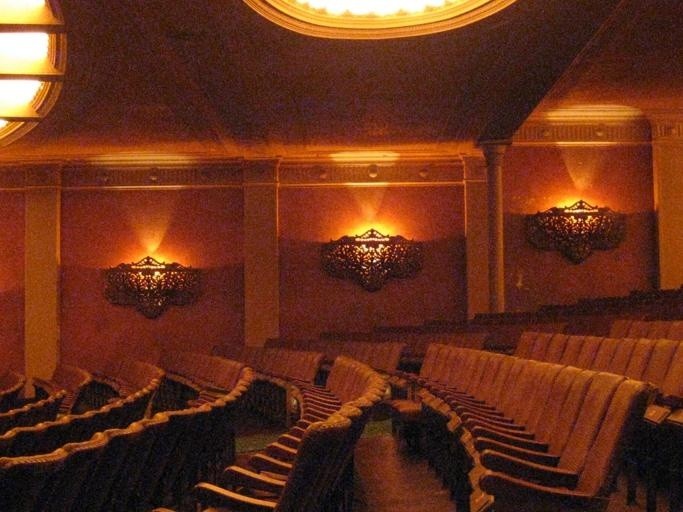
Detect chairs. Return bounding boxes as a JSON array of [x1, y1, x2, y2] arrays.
[[0, 287, 683, 512]]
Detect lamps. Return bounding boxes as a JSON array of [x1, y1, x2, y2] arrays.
[[524, 199, 627, 267], [104, 256, 203, 318], [320, 228, 422, 292]]
